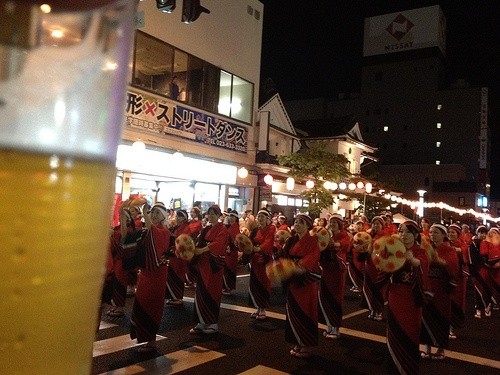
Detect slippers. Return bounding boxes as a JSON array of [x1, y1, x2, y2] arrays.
[[485, 309, 491, 316], [223, 291, 232, 296], [290, 343, 311, 358], [107, 309, 124, 317], [250, 312, 267, 319], [475, 314, 481, 319], [431, 353, 445, 360], [369, 314, 383, 320], [190, 325, 218, 335], [323, 330, 340, 339], [422, 351, 431, 358]]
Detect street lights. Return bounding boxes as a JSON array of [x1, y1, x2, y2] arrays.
[[417, 190, 427, 220], [482, 208, 488, 225], [439, 202, 444, 219]]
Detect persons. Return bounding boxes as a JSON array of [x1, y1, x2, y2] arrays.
[[168, 75, 184, 100], [174, 205, 227, 335], [271, 214, 320, 358], [119, 203, 169, 354], [371, 221, 430, 375], [102, 198, 500, 362], [233, 208, 276, 321]]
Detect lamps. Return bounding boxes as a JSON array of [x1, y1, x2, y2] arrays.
[[181, 0, 211, 24], [154, 0, 175, 13]]
